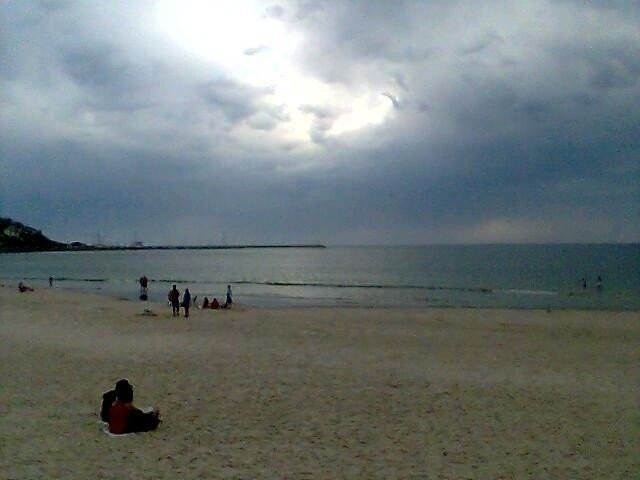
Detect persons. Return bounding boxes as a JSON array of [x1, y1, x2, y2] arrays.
[[193, 295, 199, 309], [167, 284, 181, 316], [48, 276, 53, 288], [225, 284, 233, 308], [202, 296, 209, 308], [106, 383, 163, 434], [18, 282, 34, 292], [211, 298, 219, 308], [101, 379, 158, 423], [182, 288, 192, 317]]
